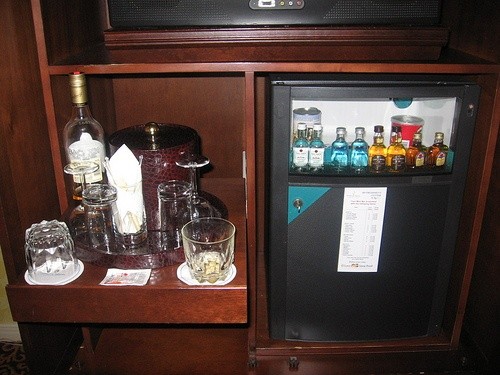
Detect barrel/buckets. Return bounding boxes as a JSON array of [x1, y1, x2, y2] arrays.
[[389, 114, 425, 149], [108, 121, 210, 232], [292, 108, 322, 140]]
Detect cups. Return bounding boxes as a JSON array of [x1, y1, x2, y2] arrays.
[[109, 182, 148, 248], [25, 219, 80, 285], [80, 182, 118, 252], [181, 216, 236, 284], [157, 180, 194, 251]]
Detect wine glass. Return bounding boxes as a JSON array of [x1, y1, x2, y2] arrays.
[[63, 162, 100, 248]]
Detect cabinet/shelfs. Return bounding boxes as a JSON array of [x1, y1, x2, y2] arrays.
[[0, 0, 499, 375]]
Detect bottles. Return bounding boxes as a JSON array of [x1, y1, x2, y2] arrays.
[[62, 71, 107, 207], [292, 123, 448, 173]]
